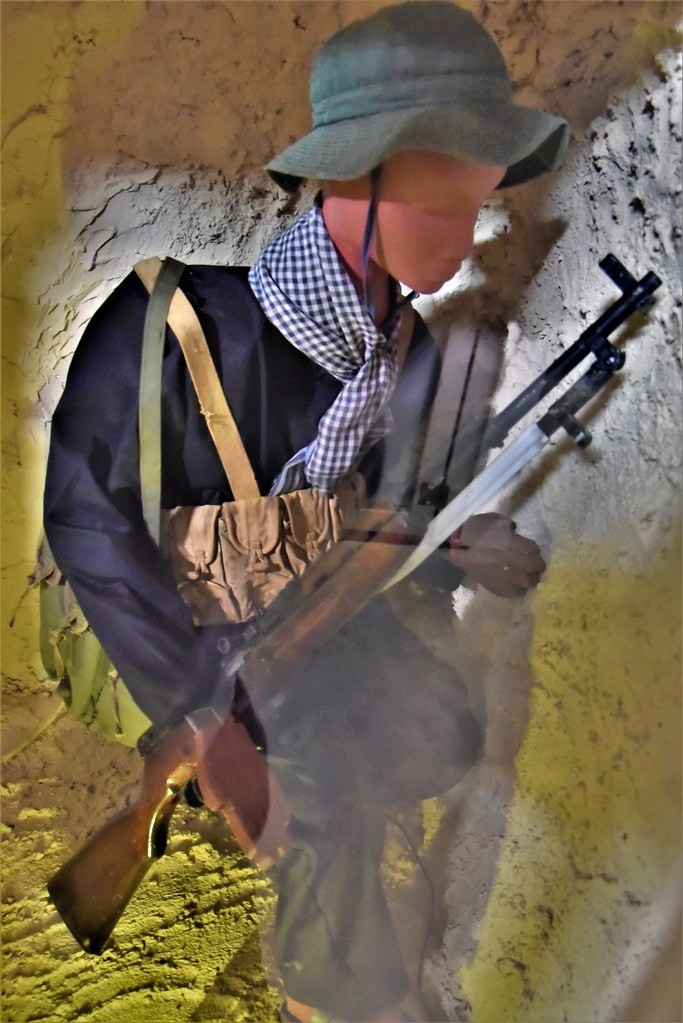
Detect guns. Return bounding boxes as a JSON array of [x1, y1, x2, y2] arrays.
[[44, 250, 664, 957]]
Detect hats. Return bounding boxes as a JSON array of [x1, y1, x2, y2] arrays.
[[264, 1, 570, 196]]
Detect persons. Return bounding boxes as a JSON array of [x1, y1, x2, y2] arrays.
[[40, 0, 571, 1021]]
